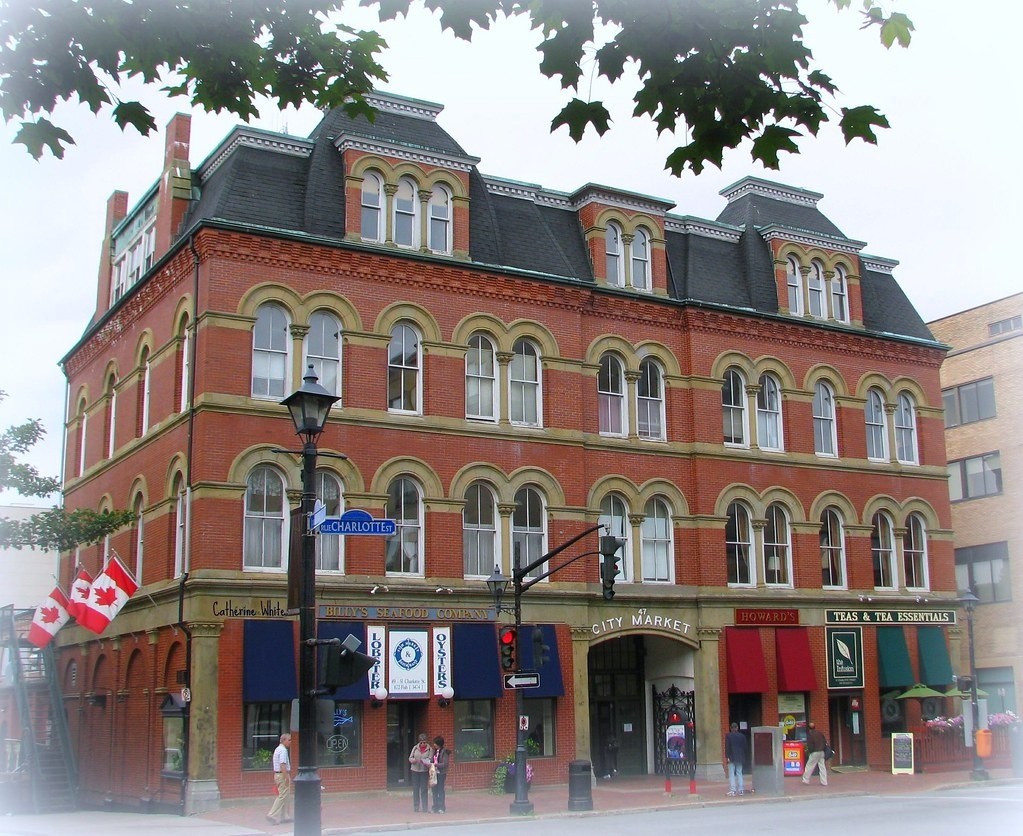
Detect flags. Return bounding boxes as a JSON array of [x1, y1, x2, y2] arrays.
[[67, 569, 93, 618], [28, 586, 72, 649], [75, 557, 141, 634]]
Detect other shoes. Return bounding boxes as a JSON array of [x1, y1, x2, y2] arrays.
[[438, 809, 443, 814], [726, 791, 735, 796], [281, 817, 294, 823], [422, 808, 428, 811], [430, 810, 437, 814], [265, 816, 279, 825], [736, 790, 743, 795], [414, 806, 419, 811]]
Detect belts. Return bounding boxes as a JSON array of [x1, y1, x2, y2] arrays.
[[276, 771, 284, 773]]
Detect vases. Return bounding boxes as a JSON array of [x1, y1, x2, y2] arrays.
[[504, 772, 531, 793], [908, 726, 964, 763], [988, 724, 1011, 754]]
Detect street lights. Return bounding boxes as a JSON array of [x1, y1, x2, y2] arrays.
[[484, 564, 534, 816], [957, 587, 990, 782], [271, 364, 348, 836]]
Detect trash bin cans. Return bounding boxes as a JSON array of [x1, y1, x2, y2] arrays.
[[567, 759, 594, 811]]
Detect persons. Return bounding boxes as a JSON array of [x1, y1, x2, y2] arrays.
[[265, 734, 293, 825], [408, 733, 452, 814], [802, 723, 827, 785], [725, 722, 748, 796]]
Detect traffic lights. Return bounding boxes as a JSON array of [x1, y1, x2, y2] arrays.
[[498, 625, 517, 670], [957, 675, 973, 693], [601, 535, 628, 601], [532, 628, 551, 666]]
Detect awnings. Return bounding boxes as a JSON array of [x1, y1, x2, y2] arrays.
[[243, 618, 296, 701], [517, 625, 564, 699], [918, 627, 953, 685], [775, 628, 817, 691], [877, 627, 914, 687], [317, 620, 369, 700], [726, 627, 769, 693], [452, 624, 503, 699]]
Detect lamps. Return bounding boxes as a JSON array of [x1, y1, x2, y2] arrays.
[[370, 585, 380, 595], [435, 588, 443, 594], [915, 598, 920, 605], [383, 586, 389, 593], [867, 597, 872, 602], [446, 589, 454, 594], [922, 598, 928, 605], [858, 597, 864, 602]]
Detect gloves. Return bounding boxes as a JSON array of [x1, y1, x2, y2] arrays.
[[413, 759, 418, 764], [436, 763, 442, 769]]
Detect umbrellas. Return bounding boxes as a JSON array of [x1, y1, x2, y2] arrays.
[[944, 686, 988, 698], [893, 683, 947, 716]]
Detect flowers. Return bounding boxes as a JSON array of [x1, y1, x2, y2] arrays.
[[924, 714, 965, 734], [987, 710, 1020, 729], [489, 753, 534, 796]]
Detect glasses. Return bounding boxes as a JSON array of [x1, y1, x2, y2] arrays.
[[420, 741, 426, 744]]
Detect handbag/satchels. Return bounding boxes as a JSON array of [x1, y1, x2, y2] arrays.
[[824, 747, 835, 760], [429, 764, 437, 788]]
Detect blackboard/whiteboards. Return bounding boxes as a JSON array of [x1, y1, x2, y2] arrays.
[[891, 732, 915, 776]]
